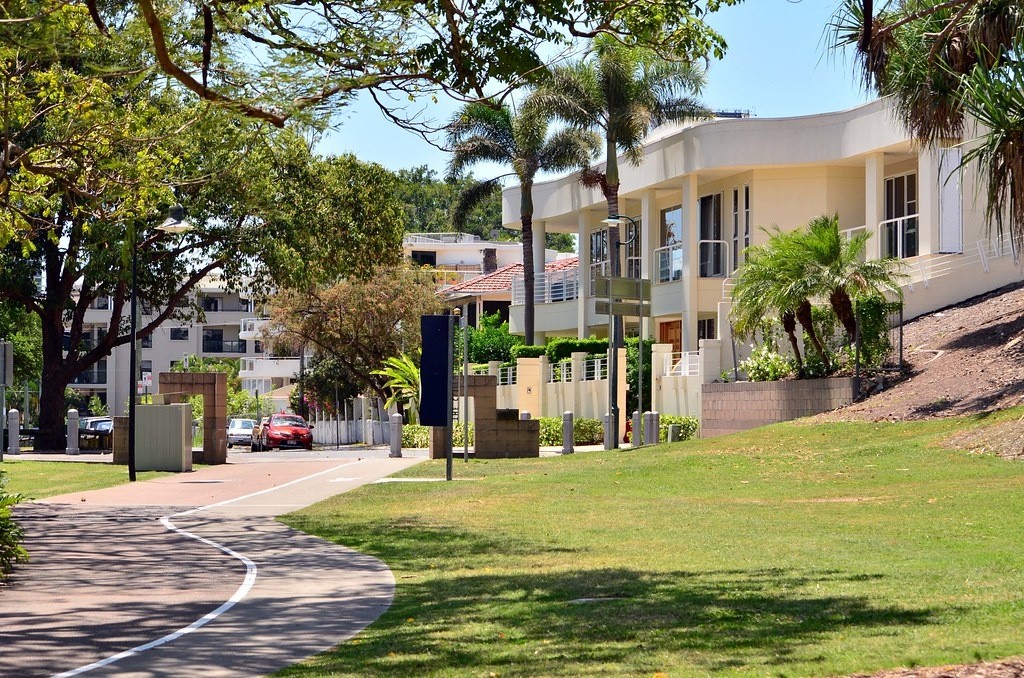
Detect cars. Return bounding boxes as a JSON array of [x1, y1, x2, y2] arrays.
[[250, 416, 269, 453], [82, 418, 114, 439], [261, 414, 314, 452], [226, 419, 256, 449]]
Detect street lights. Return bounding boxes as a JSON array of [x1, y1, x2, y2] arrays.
[[601, 215, 637, 449]]
